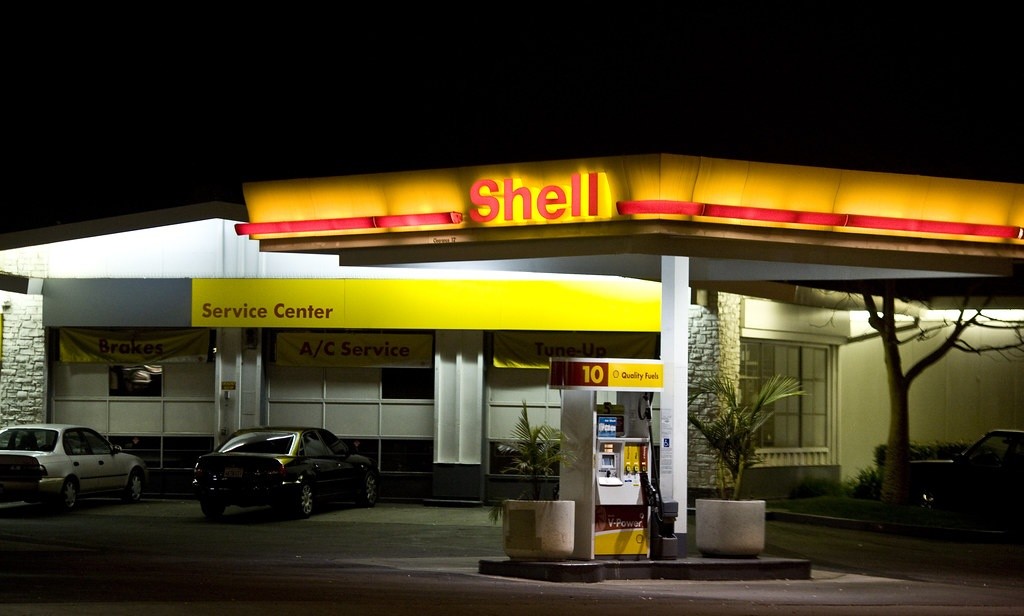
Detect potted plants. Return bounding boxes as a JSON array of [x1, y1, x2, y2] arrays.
[[690, 355, 812, 557], [874, 434, 996, 492], [486, 390, 585, 561]]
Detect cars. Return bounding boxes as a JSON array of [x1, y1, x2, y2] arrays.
[[905, 429, 1024, 544], [192, 426, 382, 519], [0, 423, 148, 514]]
